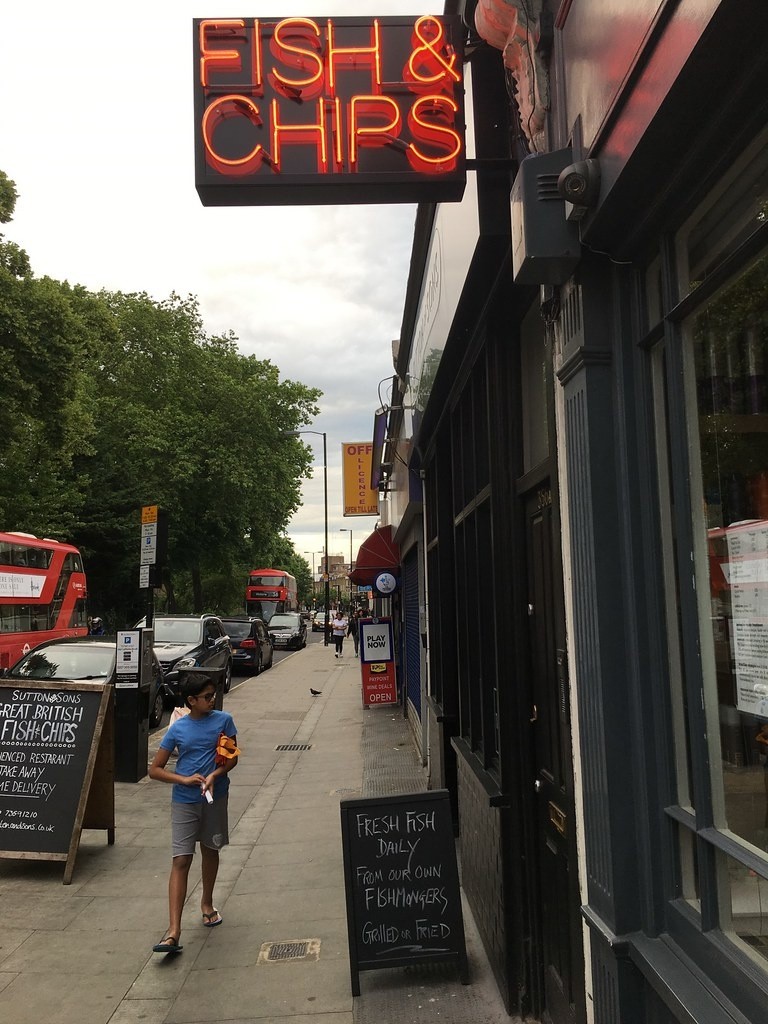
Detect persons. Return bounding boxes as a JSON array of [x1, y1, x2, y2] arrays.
[[332, 612, 347, 658], [346, 607, 370, 656], [148, 674, 238, 952]]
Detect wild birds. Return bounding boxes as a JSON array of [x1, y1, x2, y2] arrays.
[[309, 688, 323, 697]]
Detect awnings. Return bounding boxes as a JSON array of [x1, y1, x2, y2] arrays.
[[347, 524, 400, 586]]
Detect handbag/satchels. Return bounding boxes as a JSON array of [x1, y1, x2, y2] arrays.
[[215, 732, 241, 765]]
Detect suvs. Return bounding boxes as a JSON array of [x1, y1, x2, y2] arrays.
[[300, 610, 311, 620], [219, 616, 275, 674], [134, 612, 232, 702], [312, 612, 334, 632]]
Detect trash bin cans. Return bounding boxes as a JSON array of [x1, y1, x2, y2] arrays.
[[331, 628, 335, 644], [178, 667, 226, 711]]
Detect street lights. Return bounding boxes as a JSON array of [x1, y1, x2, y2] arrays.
[[304, 551, 323, 609], [333, 585, 341, 616], [340, 529, 352, 617], [285, 430, 329, 646]]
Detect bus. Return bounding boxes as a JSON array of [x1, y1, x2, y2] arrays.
[[246, 568, 298, 623], [0, 531, 92, 674]]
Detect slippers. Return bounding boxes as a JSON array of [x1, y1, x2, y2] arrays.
[[203, 906, 222, 927], [152, 937, 183, 952]]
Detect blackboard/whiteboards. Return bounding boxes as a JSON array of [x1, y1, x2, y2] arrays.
[[340, 789, 466, 970], [0, 680, 115, 861]]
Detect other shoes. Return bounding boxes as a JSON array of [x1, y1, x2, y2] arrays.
[[339, 653, 343, 658], [355, 653, 358, 657], [334, 652, 338, 658]]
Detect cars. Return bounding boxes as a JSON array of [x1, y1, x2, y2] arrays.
[[329, 610, 338, 618], [267, 611, 307, 649], [0, 637, 165, 727], [309, 609, 317, 617]]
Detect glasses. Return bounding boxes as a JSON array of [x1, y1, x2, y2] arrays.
[[193, 689, 217, 701]]
